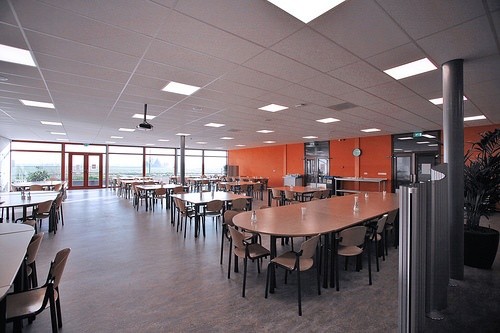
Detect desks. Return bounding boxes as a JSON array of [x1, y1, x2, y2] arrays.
[[11, 180, 65, 192], [0, 192, 60, 222], [0, 218, 36, 301], [111, 177, 269, 212], [232, 191, 399, 286], [271, 185, 325, 204], [172, 190, 253, 238]]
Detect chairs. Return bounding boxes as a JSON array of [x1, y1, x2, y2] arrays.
[[0, 182, 72, 333], [169, 187, 399, 317]]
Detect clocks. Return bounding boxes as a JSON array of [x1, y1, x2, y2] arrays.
[[353, 148, 361, 156]]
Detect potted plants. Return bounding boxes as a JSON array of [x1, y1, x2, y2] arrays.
[[461, 127, 500, 268]]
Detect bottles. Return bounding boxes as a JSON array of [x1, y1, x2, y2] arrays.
[[382, 189, 387, 200], [250, 210, 257, 223], [353, 194, 360, 211]]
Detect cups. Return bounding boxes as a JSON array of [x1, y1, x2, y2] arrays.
[[302, 207, 306, 216], [365, 192, 369, 200]]
